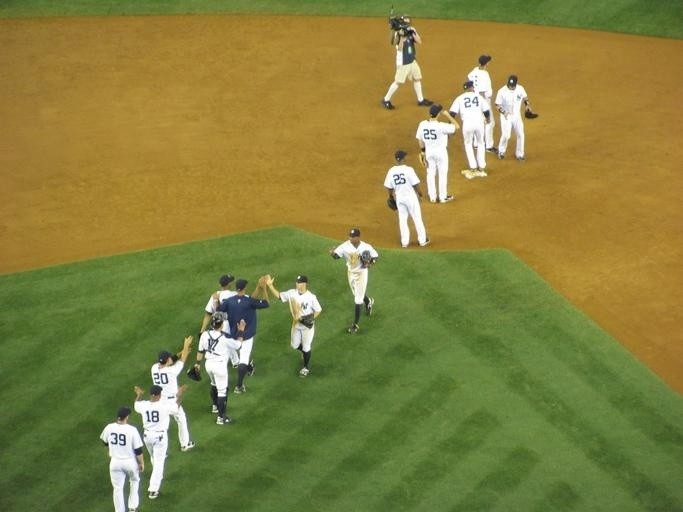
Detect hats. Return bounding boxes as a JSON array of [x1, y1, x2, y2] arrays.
[[211, 313, 223, 326], [463, 81, 473, 89], [349, 229, 360, 237], [429, 105, 441, 117], [117, 408, 130, 419], [296, 275, 306, 283], [508, 76, 517, 86], [220, 275, 247, 289], [479, 56, 491, 64], [395, 150, 406, 160], [158, 351, 169, 364], [150, 385, 162, 395]]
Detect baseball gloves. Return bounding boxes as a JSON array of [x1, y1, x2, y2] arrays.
[[298, 314, 313, 327], [526, 110, 537, 118], [361, 250, 371, 267], [387, 198, 397, 210], [419, 153, 428, 166], [187, 365, 201, 382]]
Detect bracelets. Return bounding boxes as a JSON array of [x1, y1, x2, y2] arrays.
[[236, 330, 245, 338]]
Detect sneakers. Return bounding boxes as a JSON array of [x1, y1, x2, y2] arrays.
[[441, 196, 453, 203], [418, 99, 432, 106], [383, 99, 392, 108], [348, 322, 359, 334], [211, 404, 232, 424], [234, 384, 247, 394], [149, 491, 161, 499], [420, 240, 430, 246], [366, 297, 374, 315], [232, 361, 255, 376], [180, 441, 195, 452]]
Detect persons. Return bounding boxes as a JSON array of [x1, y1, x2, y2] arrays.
[[150, 334, 196, 452], [213, 275, 270, 395], [328, 226, 379, 335], [131, 383, 191, 501], [266, 272, 322, 377], [184, 310, 247, 427], [197, 274, 267, 333], [381, 149, 431, 250], [380, 12, 434, 111], [98, 405, 146, 512], [467, 53, 499, 156], [494, 74, 536, 162], [448, 80, 492, 180], [415, 105, 461, 204]]
